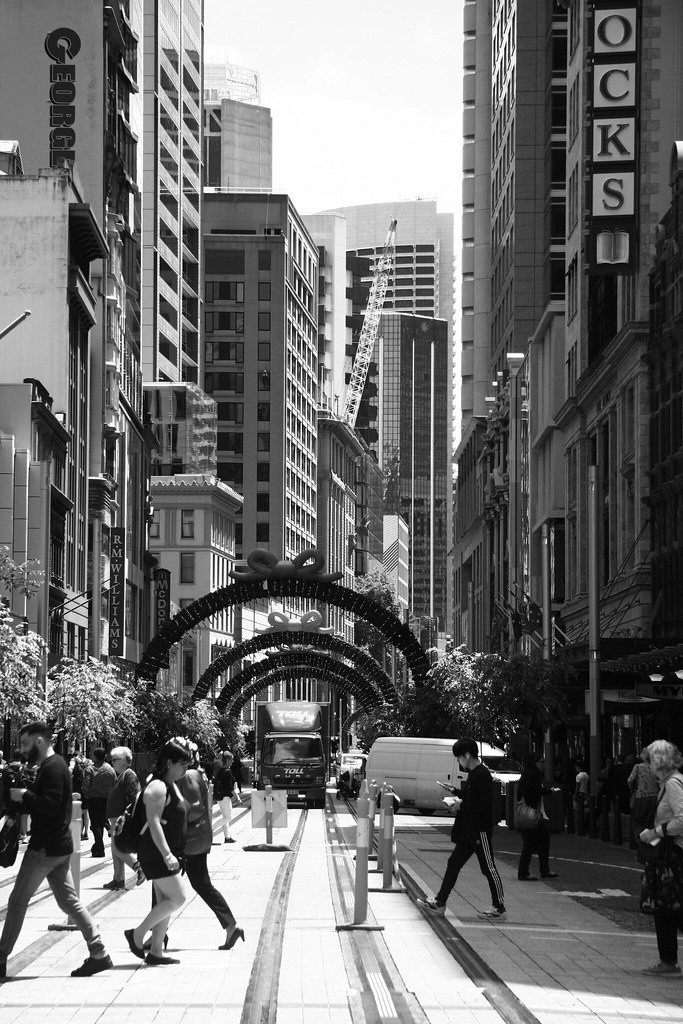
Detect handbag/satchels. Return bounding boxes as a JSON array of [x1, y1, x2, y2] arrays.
[[515, 797, 543, 829], [0, 795, 23, 868], [639, 823, 683, 915]]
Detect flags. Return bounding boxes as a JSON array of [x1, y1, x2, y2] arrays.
[[485, 590, 543, 648]]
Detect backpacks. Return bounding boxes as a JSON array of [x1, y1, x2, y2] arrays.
[[114, 776, 169, 853], [72, 756, 95, 795], [6, 763, 29, 788]]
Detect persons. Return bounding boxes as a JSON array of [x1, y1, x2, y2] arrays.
[[417, 738, 507, 920], [231, 755, 243, 793], [67, 748, 118, 857], [0, 748, 39, 844], [103, 746, 146, 888], [212, 750, 242, 843], [143, 737, 245, 951], [638, 739, 683, 977], [555, 750, 660, 849], [0, 723, 113, 981], [517, 752, 558, 881], [124, 743, 191, 965]]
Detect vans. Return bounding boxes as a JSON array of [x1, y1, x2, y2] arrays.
[[363, 735, 525, 816], [333, 753, 368, 788]]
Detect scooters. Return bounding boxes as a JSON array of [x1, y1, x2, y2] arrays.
[[335, 780, 360, 800]]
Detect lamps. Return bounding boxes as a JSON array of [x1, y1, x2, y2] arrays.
[[649, 674, 664, 681], [674, 669, 683, 679]]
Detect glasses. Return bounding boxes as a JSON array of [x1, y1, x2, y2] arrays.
[[109, 758, 124, 763], [538, 760, 545, 764]]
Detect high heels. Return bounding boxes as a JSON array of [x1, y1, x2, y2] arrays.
[[219, 927, 245, 950], [143, 935, 168, 950]]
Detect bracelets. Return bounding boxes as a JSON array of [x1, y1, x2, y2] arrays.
[[654, 828, 660, 838], [164, 853, 172, 859]]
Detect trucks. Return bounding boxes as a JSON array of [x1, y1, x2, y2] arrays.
[[253, 700, 330, 810]]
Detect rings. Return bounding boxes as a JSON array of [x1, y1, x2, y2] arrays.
[[170, 868, 173, 870]]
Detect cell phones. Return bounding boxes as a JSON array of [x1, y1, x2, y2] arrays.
[[437, 780, 454, 791], [640, 828, 661, 846]]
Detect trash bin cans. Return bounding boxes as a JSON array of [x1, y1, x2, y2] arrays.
[[505, 781, 520, 830]]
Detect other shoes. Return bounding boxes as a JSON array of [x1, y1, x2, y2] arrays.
[[70, 954, 113, 977], [417, 897, 446, 917], [0, 962, 8, 981], [518, 876, 538, 881], [541, 872, 558, 879], [477, 905, 507, 920], [144, 954, 180, 966], [125, 929, 145, 959], [14, 822, 237, 891], [642, 959, 682, 977]]
